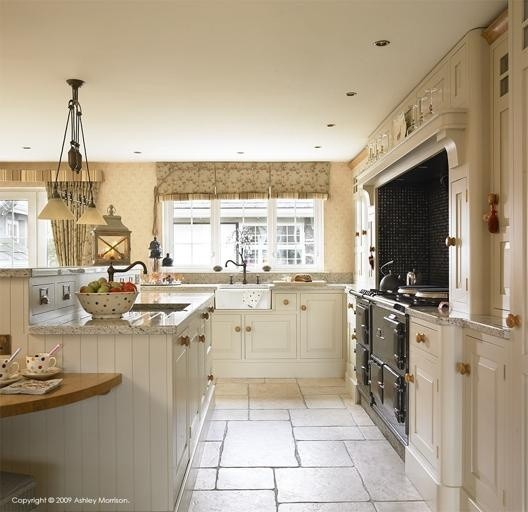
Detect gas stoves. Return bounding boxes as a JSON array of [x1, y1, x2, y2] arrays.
[[359, 287, 447, 306]]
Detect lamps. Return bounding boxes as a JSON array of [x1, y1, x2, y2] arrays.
[[36, 77, 109, 227], [87, 203, 131, 267]]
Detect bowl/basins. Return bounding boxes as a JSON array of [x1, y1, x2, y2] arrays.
[[74, 290, 141, 320]]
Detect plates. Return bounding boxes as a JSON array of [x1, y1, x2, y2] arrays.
[[0, 373, 22, 385], [20, 366, 63, 380]]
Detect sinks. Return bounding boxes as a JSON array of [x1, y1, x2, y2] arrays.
[[131, 304, 190, 313], [215, 284, 272, 310]]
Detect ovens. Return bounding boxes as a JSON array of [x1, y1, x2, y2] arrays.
[[349, 288, 409, 450]]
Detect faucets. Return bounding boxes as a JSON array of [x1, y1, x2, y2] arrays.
[[225, 253, 248, 284], [108, 261, 147, 282]]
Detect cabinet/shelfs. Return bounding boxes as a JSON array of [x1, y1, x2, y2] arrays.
[[297, 286, 346, 380], [0, 264, 217, 512], [210, 284, 298, 379], [343, 0, 527, 512]]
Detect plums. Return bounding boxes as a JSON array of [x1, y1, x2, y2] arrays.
[[80, 278, 121, 293]]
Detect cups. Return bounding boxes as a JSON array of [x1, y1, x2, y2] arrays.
[[406, 268, 422, 286], [26, 351, 57, 372], [0, 358, 19, 379]]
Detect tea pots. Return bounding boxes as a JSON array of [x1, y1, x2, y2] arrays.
[[378, 260, 406, 292]]
